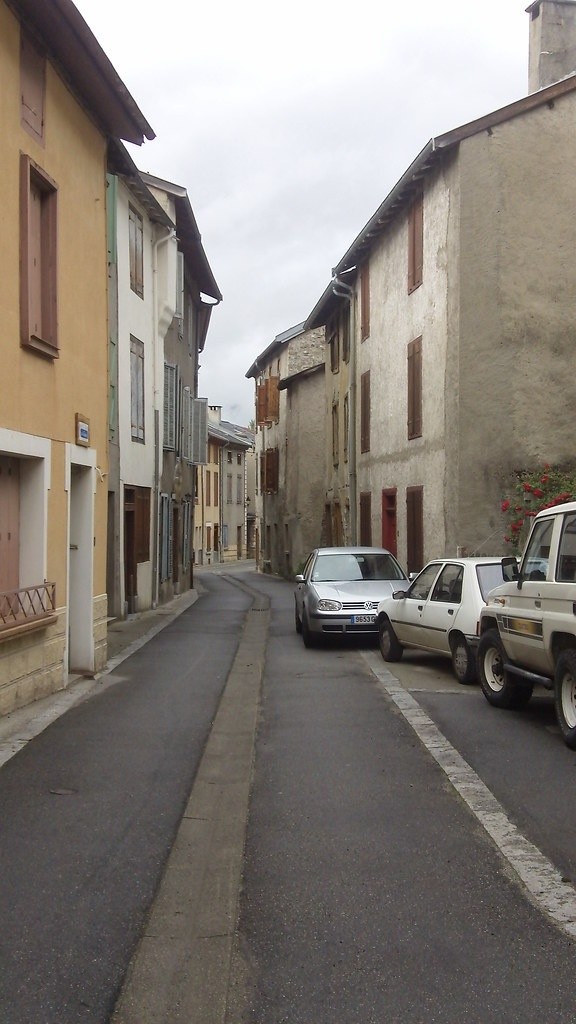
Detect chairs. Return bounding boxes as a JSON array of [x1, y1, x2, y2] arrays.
[[363, 559, 387, 577], [449, 579, 462, 602]]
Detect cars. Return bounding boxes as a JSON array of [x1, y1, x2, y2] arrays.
[[293, 546, 420, 650], [373, 556, 548, 686]]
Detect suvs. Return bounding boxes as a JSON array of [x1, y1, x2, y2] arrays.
[[476, 502, 576, 749]]
